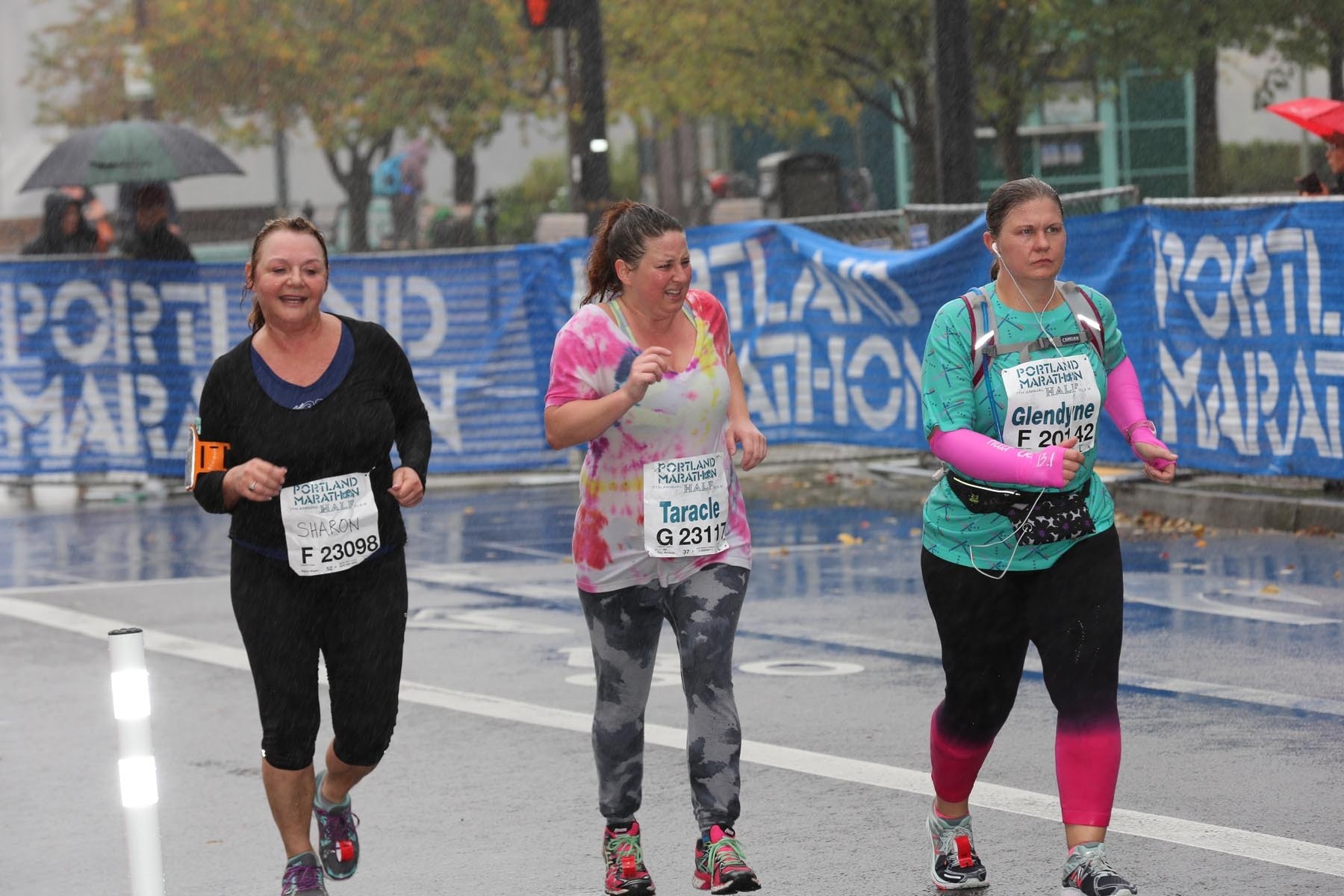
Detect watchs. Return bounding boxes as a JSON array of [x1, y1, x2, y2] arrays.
[[1126, 420, 1157, 444]]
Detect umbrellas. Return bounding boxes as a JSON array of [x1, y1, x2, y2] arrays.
[[1266, 96, 1344, 137], [16, 119, 246, 193]]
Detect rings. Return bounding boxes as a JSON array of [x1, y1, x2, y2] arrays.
[[248, 481, 256, 490]]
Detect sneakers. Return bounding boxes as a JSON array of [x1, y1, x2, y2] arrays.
[[1061, 845, 1138, 895], [312, 771, 362, 881], [926, 798, 991, 892], [694, 824, 761, 894], [281, 851, 328, 896], [603, 822, 655, 896]]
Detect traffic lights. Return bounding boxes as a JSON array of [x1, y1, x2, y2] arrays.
[[518, 0, 570, 30]]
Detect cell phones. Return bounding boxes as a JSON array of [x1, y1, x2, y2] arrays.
[[184, 429, 196, 490]]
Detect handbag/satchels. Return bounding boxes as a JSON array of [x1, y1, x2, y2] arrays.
[[1007, 499, 1096, 547]]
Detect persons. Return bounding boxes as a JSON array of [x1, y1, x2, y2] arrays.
[[198, 218, 432, 896], [22, 177, 194, 262], [707, 165, 877, 217], [332, 138, 430, 252], [541, 198, 767, 896], [919, 176, 1178, 896], [1294, 131, 1344, 197]]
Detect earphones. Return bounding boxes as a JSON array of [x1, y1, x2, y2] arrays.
[[991, 243, 1002, 258]]
